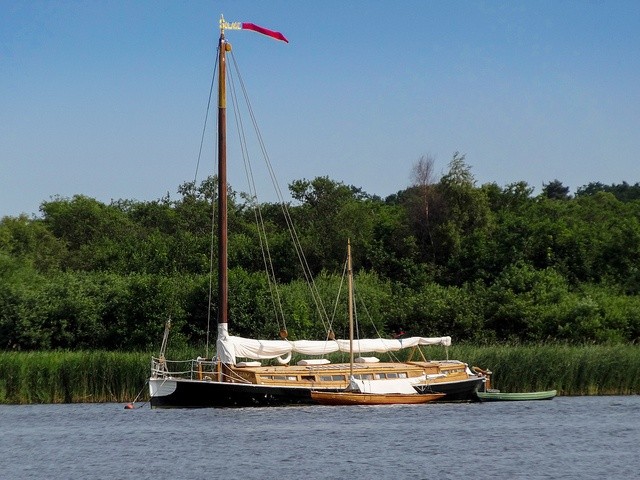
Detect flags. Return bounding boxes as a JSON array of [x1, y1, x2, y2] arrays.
[[221, 18, 287, 42]]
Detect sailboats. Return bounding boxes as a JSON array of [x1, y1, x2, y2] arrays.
[[311, 237, 448, 405]]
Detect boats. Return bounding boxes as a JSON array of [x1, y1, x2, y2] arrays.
[[476, 389, 559, 401], [150, 14, 495, 410]]
